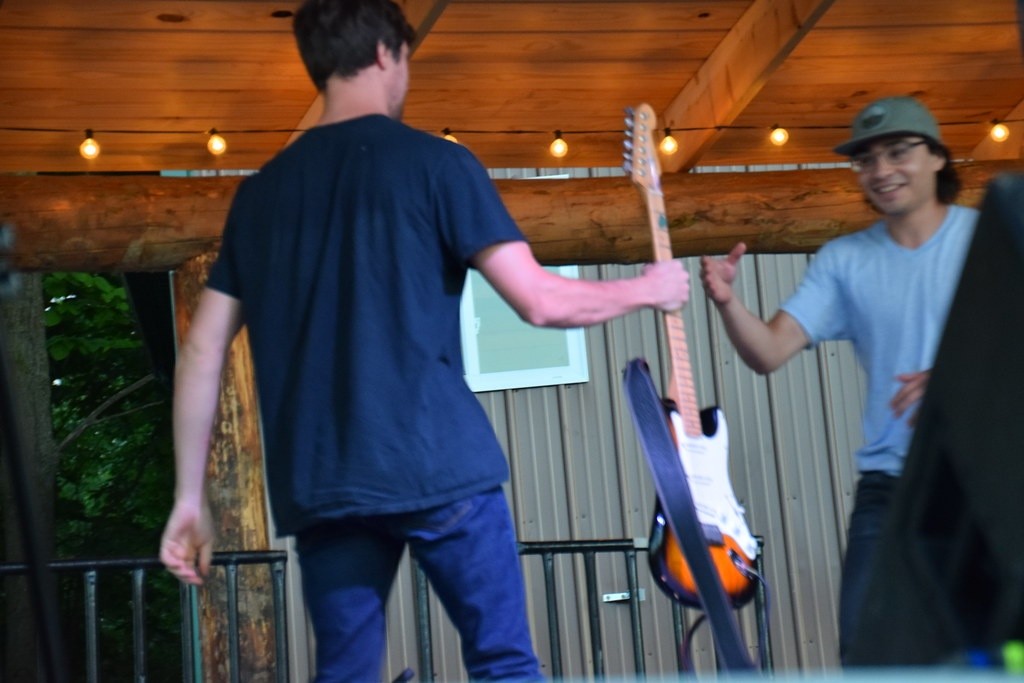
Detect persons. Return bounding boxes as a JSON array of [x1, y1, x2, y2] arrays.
[[700, 98, 980, 670], [888, 0, 1024, 672], [154, 1, 690, 681]]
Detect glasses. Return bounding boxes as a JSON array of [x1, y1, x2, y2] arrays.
[[849, 136, 931, 173]]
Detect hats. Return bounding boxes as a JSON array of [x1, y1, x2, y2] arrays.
[[832, 95, 941, 154]]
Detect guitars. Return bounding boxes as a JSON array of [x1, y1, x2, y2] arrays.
[[620, 100, 761, 610]]
